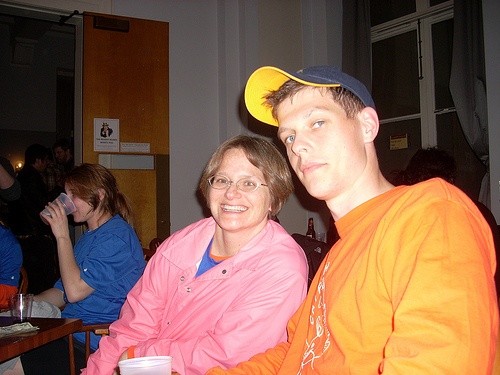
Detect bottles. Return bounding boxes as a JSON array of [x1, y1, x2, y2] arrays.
[[306, 218, 316, 240]]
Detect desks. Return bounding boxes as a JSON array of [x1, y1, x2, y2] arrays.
[[0, 315, 83, 375]]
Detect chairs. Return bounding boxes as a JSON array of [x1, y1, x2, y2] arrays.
[[80, 238, 163, 366]]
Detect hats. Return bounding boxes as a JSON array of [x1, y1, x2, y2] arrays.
[[244, 64, 376, 127]]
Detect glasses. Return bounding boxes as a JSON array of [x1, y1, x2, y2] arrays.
[[207, 174, 269, 193]]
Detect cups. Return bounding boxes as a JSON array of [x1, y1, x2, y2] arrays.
[[40, 192, 77, 225], [118, 356, 173, 375], [9, 293, 34, 326]]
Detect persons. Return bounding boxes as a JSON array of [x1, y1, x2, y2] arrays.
[[0, 158, 35, 214], [0, 220, 24, 312], [76, 133, 309, 375], [42, 141, 74, 189], [19, 143, 50, 198], [0, 154, 147, 375], [199, 66, 500, 375]]
